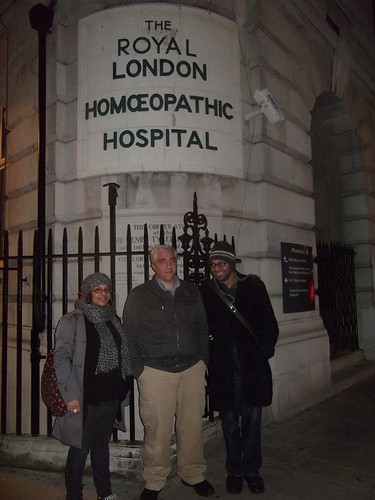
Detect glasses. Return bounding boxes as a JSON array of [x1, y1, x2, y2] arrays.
[[91, 288, 112, 294], [209, 261, 227, 268]]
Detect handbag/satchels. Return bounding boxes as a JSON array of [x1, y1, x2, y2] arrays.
[[40, 349, 69, 417]]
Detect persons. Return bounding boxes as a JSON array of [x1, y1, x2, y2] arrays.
[[199, 241, 279, 494], [51, 272, 134, 500], [122, 245, 215, 500]]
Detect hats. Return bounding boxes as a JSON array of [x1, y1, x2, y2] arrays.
[[208, 241, 236, 264], [79, 273, 112, 303]]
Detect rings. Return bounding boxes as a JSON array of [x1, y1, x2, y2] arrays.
[[72, 409, 78, 413]]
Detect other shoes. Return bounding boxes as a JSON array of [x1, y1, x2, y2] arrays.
[[244, 476, 265, 494], [97, 492, 118, 500], [140, 487, 163, 500], [180, 478, 215, 497], [226, 474, 243, 494]]
